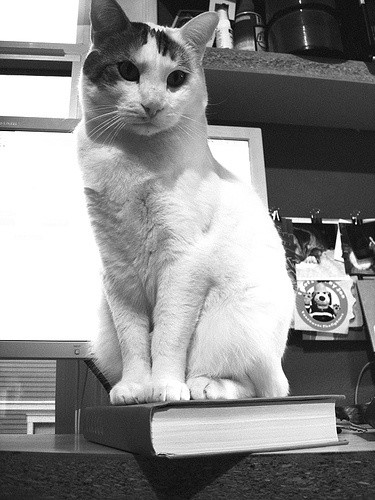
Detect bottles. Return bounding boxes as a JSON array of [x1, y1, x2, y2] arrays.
[[215, 3, 235, 49], [270, 208, 283, 234], [310, 209, 335, 252], [232, 0, 268, 52], [349, 210, 373, 259]]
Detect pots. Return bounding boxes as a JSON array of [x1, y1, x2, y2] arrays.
[[266, 3, 347, 59]]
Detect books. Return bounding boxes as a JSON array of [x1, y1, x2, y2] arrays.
[[80, 394, 350, 460]]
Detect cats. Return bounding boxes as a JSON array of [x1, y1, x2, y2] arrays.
[[67, 0, 297, 407]]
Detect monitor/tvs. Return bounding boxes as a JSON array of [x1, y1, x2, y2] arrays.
[[0, 115, 268, 365]]
